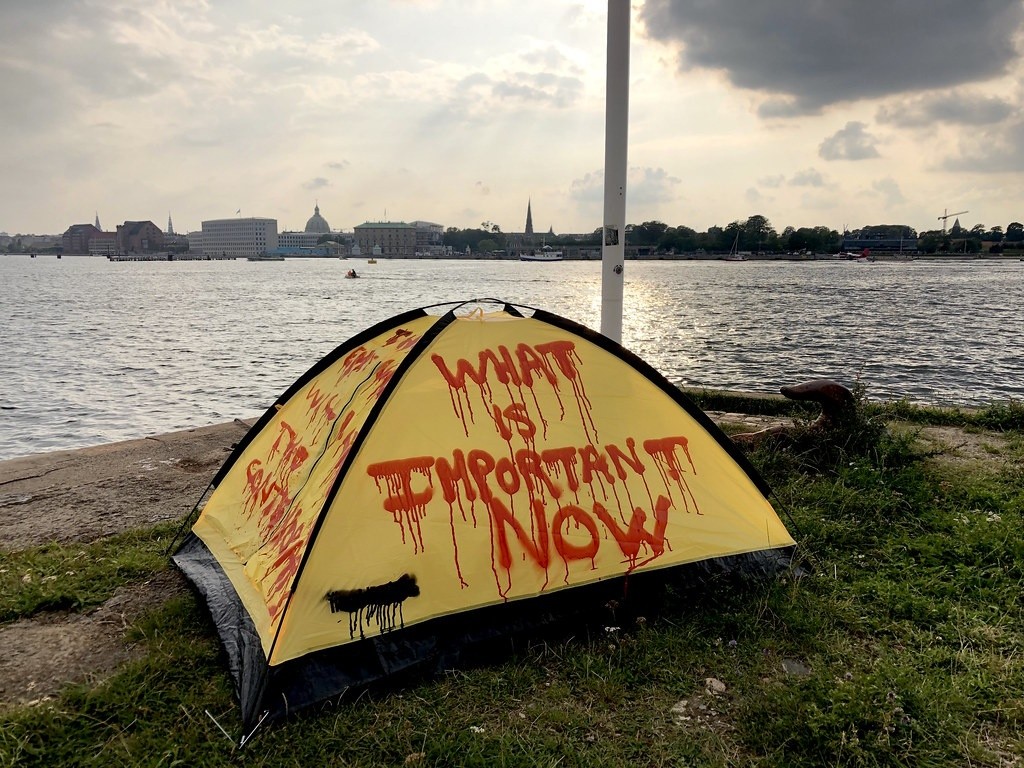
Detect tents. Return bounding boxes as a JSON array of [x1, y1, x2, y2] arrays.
[[165, 294, 824, 753]]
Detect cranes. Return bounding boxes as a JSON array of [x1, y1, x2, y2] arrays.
[[938, 209, 969, 237]]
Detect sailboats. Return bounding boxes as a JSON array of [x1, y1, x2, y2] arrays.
[[724, 229, 749, 261]]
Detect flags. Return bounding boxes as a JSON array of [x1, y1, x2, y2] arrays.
[[236, 209, 240, 214]]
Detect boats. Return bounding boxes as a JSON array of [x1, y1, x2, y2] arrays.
[[339, 256, 348, 260], [858, 257, 869, 262], [248, 253, 285, 261], [841, 231, 919, 255], [833, 252, 860, 260], [520, 246, 563, 260], [345, 269, 359, 279]]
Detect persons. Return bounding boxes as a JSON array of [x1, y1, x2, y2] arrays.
[[351, 269, 356, 277]]
